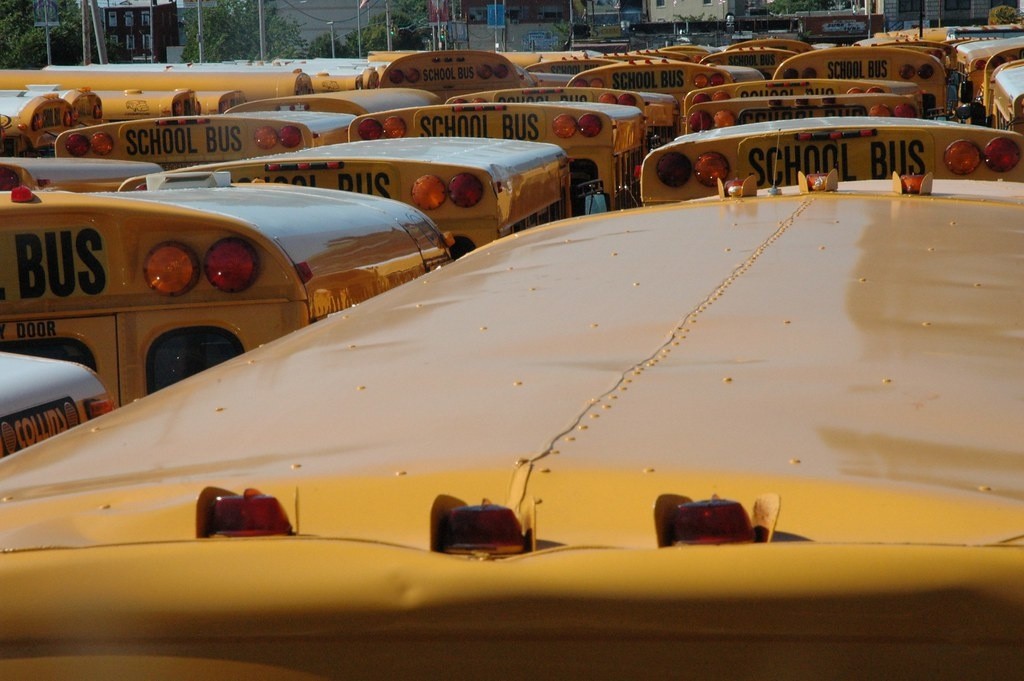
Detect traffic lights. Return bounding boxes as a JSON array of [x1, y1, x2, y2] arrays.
[[391, 26, 394, 35], [441, 30, 444, 39]]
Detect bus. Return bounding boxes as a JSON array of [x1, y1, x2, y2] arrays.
[[1, 25, 1024, 681]]
